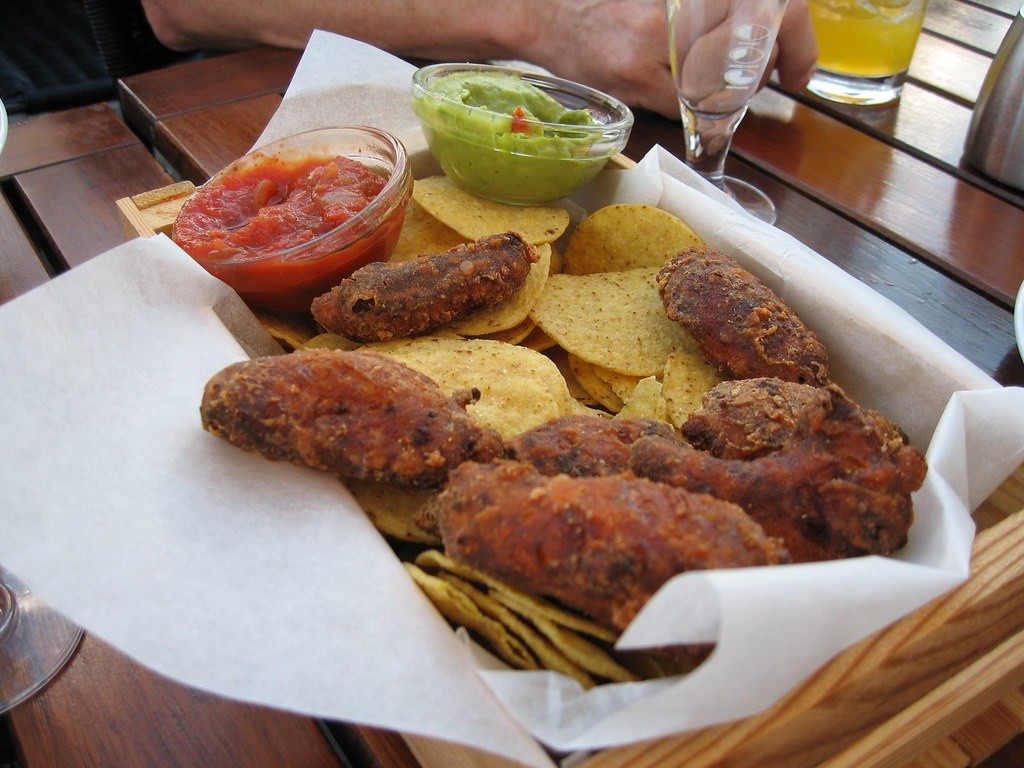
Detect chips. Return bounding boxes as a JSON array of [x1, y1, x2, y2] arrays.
[[256, 174, 739, 684]]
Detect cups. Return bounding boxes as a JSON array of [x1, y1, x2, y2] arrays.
[[806, 1, 929, 105]]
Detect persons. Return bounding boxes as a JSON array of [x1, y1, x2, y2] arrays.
[[0, 0, 818, 131]]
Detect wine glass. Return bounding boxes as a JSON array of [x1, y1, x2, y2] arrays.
[[1, 565, 85, 715], [666, 1, 790, 226]]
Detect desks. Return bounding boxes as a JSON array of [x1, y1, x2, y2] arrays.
[[0, 0, 1024, 768]]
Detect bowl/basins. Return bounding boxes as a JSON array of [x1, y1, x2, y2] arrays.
[[411, 63, 634, 207], [171, 125, 413, 311]]
